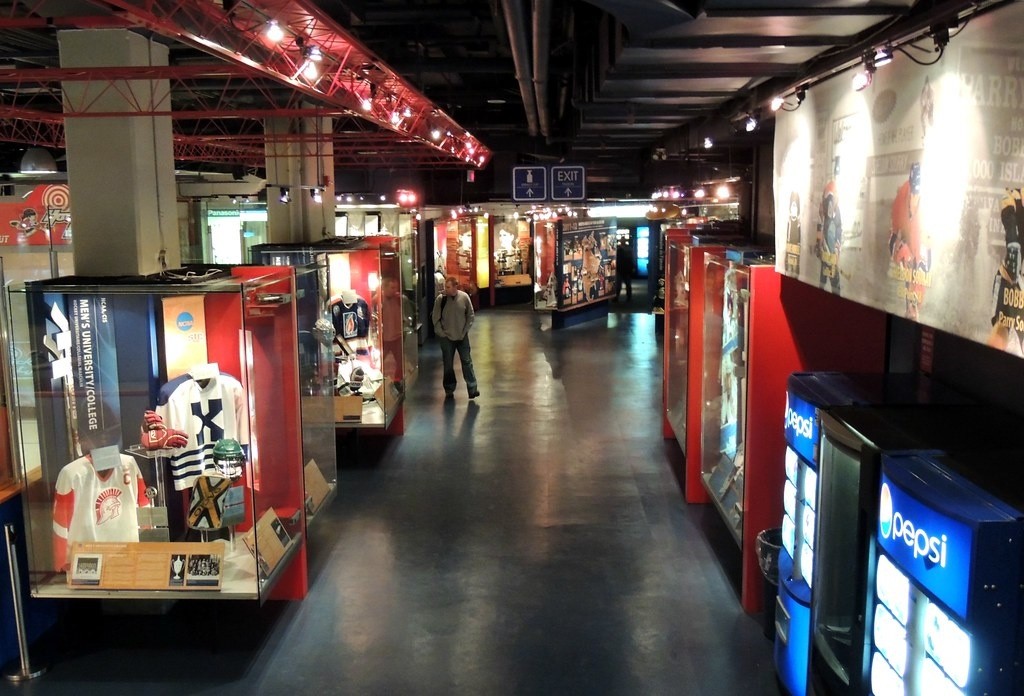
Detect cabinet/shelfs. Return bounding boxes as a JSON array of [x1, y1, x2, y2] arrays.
[[1, 215, 1024, 696]]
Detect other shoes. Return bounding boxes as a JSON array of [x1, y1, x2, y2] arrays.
[[469, 391, 480, 399], [446, 392, 454, 400]]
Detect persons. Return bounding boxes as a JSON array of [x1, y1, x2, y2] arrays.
[[616, 236, 637, 300], [432, 277, 480, 399]]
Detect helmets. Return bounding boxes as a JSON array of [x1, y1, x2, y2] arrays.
[[212, 438, 246, 467], [909, 162, 920, 194]]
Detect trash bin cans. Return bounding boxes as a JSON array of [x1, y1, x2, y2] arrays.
[[754, 526, 782, 641]]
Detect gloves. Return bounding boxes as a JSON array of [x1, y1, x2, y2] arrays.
[[141, 429, 189, 451], [141, 410, 167, 435]]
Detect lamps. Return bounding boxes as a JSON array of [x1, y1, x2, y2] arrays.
[[20, 147, 58, 174], [745, 109, 761, 132], [852, 49, 893, 91], [309, 189, 322, 203], [771, 88, 805, 112], [280, 187, 289, 204]]
